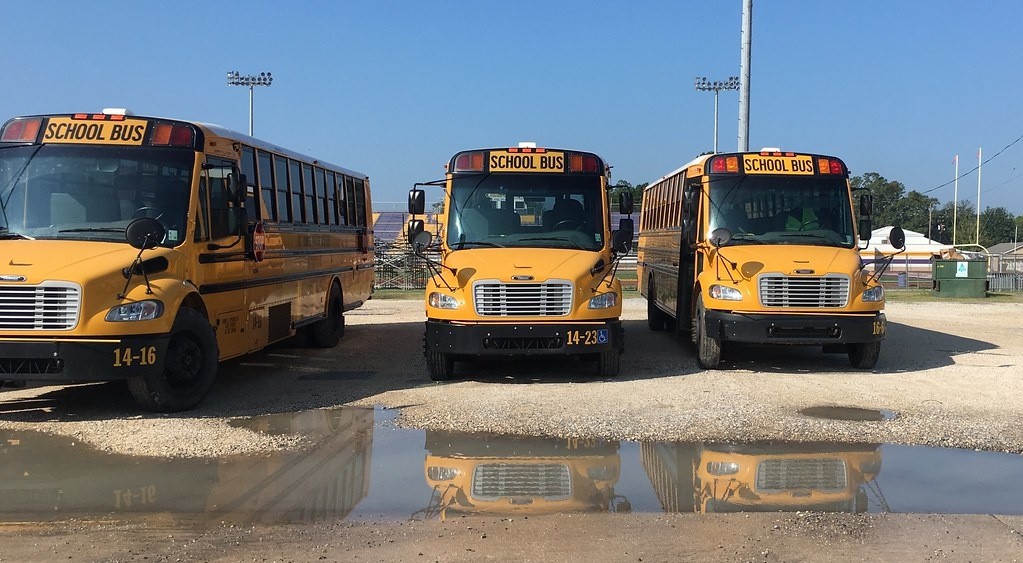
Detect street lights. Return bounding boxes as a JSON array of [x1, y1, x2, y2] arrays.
[[694, 76, 740, 154], [226, 71, 274, 136]]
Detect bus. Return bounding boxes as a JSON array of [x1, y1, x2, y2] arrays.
[[634, 150, 907, 371], [406, 145, 645, 382], [0, 108, 376, 416], [415, 423, 630, 516], [0, 405, 376, 527], [638, 433, 883, 514]]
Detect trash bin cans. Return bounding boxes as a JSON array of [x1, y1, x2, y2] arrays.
[[898, 272, 906, 288], [929, 254, 988, 297]]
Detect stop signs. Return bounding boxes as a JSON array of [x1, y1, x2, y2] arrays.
[[251, 221, 269, 264]]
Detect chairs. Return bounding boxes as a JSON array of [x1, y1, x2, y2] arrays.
[[718, 193, 844, 232], [469, 198, 584, 236]]
